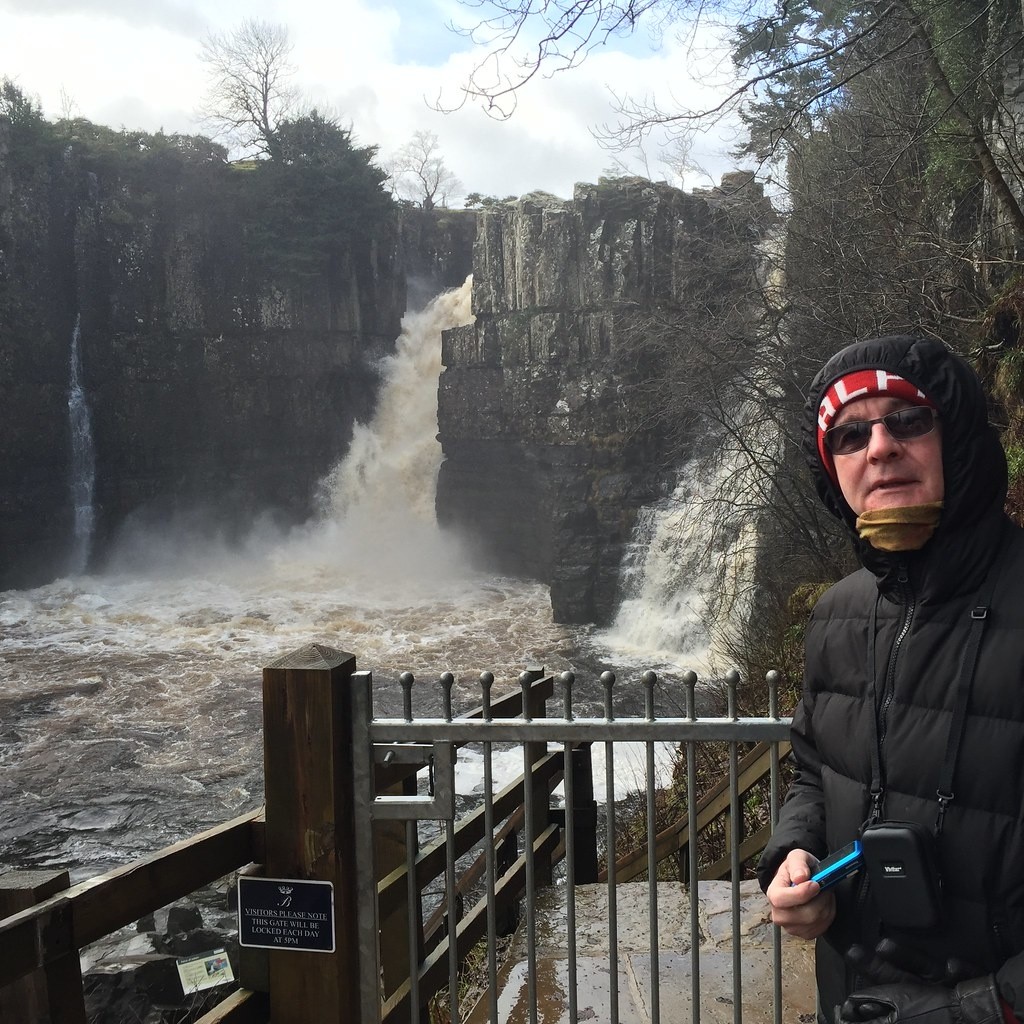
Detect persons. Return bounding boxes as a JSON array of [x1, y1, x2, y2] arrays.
[[755, 336, 1024, 1024]]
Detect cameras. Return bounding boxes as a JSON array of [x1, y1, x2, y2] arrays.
[[789, 840, 866, 894]]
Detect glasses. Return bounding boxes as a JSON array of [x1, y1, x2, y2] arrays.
[[822, 406, 940, 455]]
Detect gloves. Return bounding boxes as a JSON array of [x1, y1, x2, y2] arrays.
[[834, 939, 1006, 1024]]
